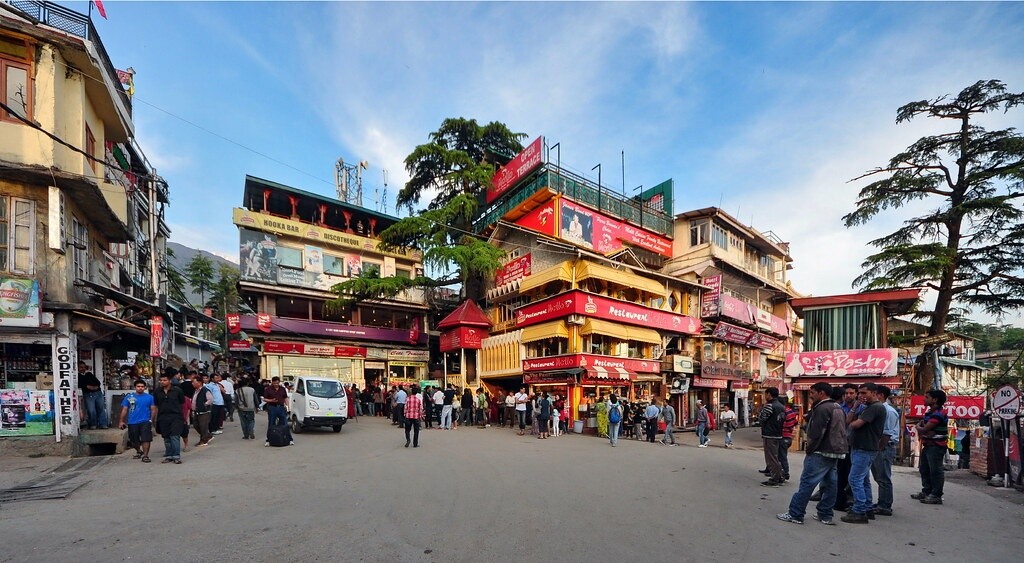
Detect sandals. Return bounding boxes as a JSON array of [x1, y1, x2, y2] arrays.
[[142, 456, 151, 463], [133, 450, 144, 459]]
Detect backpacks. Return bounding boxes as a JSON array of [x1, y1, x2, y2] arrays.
[[608, 405, 620, 423], [270, 424, 288, 446]]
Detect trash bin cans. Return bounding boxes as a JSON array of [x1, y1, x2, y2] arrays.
[[574, 420, 584, 434]]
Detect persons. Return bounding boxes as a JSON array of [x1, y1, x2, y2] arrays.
[[569, 214, 582, 238], [696, 399, 744, 448], [76, 358, 295, 464], [758, 379, 899, 525], [343, 381, 677, 447], [911, 390, 948, 504]]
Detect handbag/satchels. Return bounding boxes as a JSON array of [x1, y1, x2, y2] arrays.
[[483, 401, 488, 409], [452, 401, 461, 409], [534, 404, 542, 416]]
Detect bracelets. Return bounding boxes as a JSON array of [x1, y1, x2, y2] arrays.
[[850, 409, 856, 413]]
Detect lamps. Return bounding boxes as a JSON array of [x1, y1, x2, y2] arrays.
[[67, 241, 86, 251]]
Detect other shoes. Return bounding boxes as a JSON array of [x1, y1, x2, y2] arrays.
[[195, 441, 208, 446], [725, 444, 729, 447], [391, 420, 676, 448], [250, 433, 255, 439], [265, 442, 269, 446], [704, 438, 710, 445], [174, 459, 182, 464], [699, 444, 707, 448], [207, 435, 214, 443], [162, 458, 173, 462], [242, 436, 248, 439], [101, 426, 108, 429], [290, 441, 294, 444], [91, 426, 96, 429], [729, 442, 732, 446], [211, 430, 223, 435]]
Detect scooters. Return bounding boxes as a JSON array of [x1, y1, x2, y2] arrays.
[[198, 368, 206, 375]]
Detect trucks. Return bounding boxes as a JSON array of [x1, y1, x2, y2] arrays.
[[287, 376, 349, 434]]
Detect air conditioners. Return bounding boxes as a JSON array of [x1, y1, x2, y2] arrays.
[[567, 314, 586, 326]]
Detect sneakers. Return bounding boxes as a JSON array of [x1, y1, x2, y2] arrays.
[[758, 469, 942, 524]]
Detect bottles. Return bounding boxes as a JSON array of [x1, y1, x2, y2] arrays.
[[0, 277, 34, 318]]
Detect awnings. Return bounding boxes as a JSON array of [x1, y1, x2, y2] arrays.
[[519, 260, 573, 293], [575, 260, 666, 298], [520, 319, 569, 338], [578, 317, 661, 344], [583, 365, 637, 380], [72, 278, 221, 348]]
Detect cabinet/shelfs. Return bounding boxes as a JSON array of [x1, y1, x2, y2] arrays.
[[0, 343, 7, 389]]
